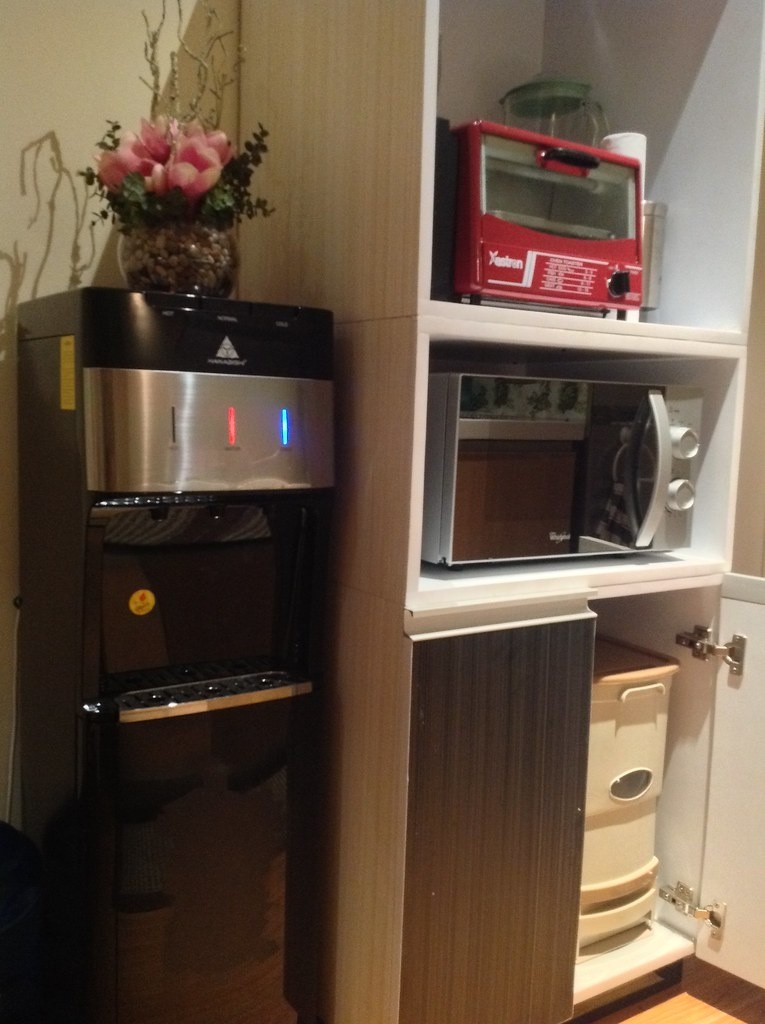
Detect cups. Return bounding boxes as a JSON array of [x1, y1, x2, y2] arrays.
[[499, 76, 610, 149]]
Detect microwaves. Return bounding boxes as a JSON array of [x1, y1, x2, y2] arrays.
[[418, 369, 703, 570]]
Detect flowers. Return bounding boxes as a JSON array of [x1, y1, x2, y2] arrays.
[[73, 1, 281, 238]]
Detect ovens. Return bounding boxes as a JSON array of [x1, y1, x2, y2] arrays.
[[438, 119, 648, 322]]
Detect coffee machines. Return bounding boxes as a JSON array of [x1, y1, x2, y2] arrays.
[[16, 284, 334, 1023]]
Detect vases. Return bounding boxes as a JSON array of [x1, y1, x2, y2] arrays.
[[118, 216, 240, 301]]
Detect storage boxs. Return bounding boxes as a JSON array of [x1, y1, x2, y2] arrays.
[[582, 623, 681, 820]]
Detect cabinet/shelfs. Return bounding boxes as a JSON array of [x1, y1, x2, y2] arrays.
[[321, 573, 765, 1024], [236, 0, 765, 602]]
